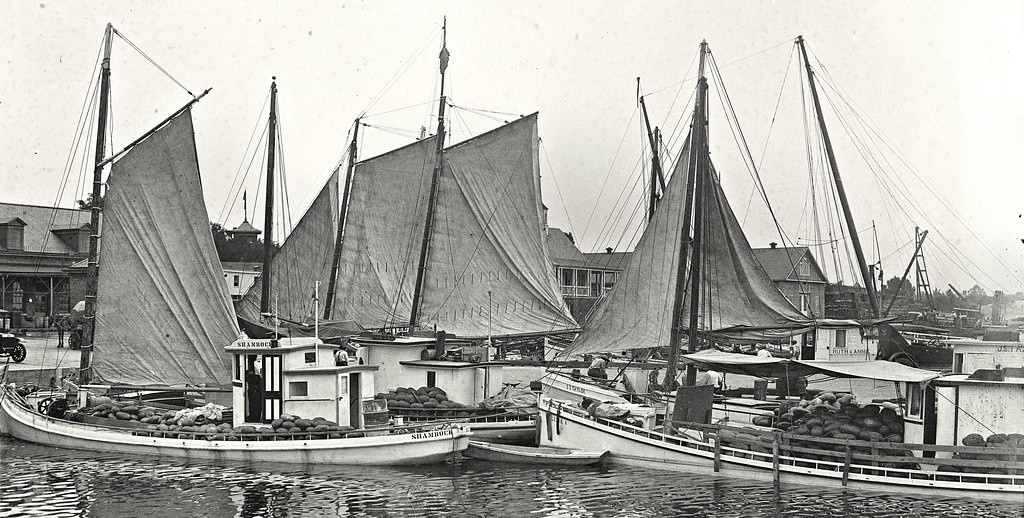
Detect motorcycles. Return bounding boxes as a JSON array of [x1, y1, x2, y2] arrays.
[[0, 333, 26, 363]]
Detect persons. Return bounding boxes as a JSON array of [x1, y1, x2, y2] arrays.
[[334, 344, 350, 367], [704, 364, 722, 388], [587, 355, 611, 380], [757, 344, 774, 358]]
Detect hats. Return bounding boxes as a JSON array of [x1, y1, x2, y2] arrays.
[[599, 354, 610, 362], [338, 342, 349, 348], [760, 344, 767, 348], [790, 340, 797, 343]]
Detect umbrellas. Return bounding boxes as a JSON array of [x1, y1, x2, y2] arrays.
[[72, 300, 96, 312]]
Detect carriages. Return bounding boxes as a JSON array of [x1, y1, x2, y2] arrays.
[[47, 301, 96, 351]]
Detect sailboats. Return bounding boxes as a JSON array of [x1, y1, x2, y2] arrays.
[[0, 13, 1024, 504]]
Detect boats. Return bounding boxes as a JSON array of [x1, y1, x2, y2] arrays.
[[461, 440, 610, 466]]
